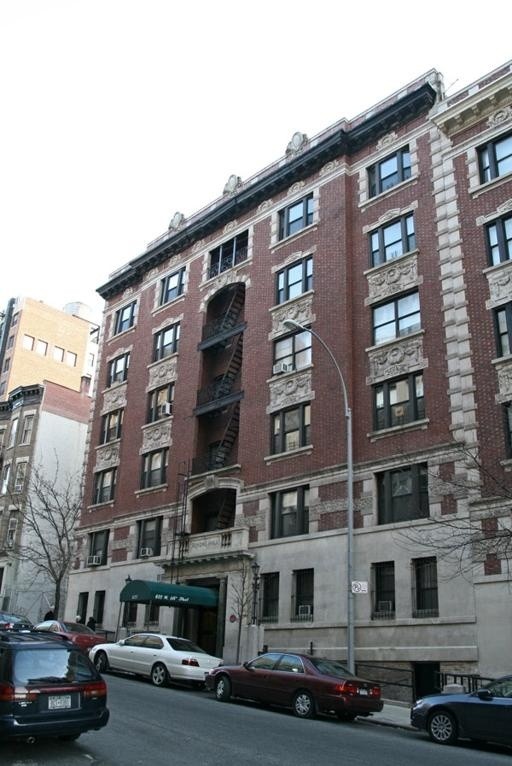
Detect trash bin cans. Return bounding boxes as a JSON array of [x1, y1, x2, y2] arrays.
[[411, 662, 441, 702]]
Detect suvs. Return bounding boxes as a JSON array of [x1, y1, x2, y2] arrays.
[[0, 626, 111, 747]]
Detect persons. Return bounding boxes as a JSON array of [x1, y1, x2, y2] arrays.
[[257, 645, 268, 656], [87, 617, 96, 632], [75, 615, 83, 624], [44, 610, 57, 621]]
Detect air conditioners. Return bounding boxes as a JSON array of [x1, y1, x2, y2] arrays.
[[272, 363, 287, 376], [141, 548, 153, 556], [161, 402, 172, 416], [87, 555, 102, 565]]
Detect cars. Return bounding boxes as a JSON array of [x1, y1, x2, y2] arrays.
[[201, 651, 385, 722], [410, 672, 512, 752], [31, 619, 107, 660], [86, 632, 225, 687], [0, 610, 33, 632]]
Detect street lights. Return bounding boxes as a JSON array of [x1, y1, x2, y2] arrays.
[[116, 574, 132, 640], [238, 560, 265, 663], [283, 317, 358, 678]]
